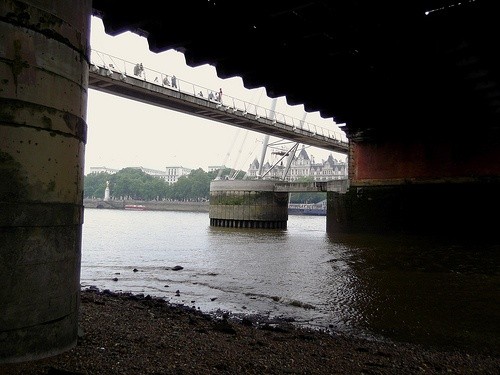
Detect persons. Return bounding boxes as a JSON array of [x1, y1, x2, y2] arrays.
[[199, 88, 223, 103], [163, 75, 178, 89], [133, 62, 143, 77]]
